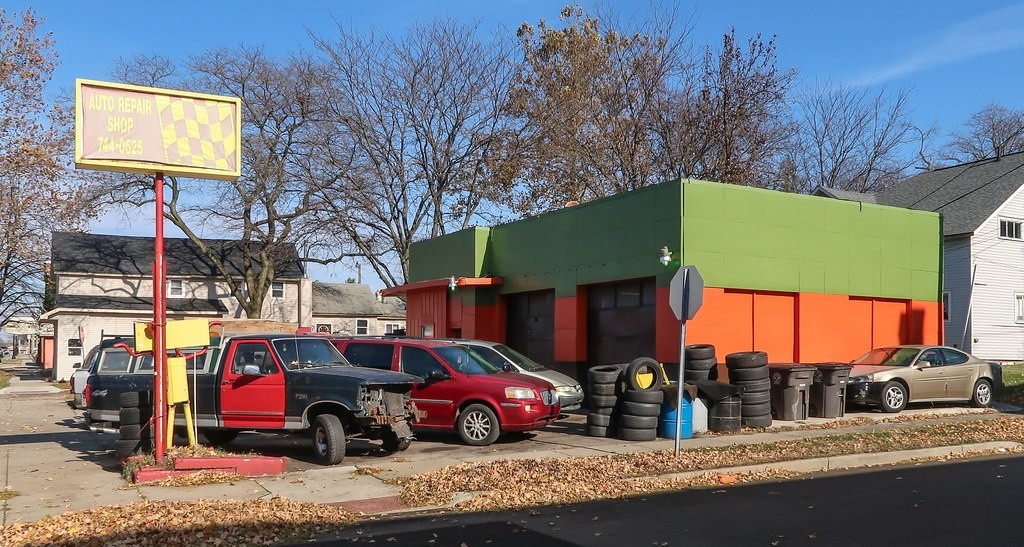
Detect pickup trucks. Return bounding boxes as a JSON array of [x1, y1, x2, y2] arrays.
[[70, 369, 89, 395], [330, 339, 559, 446], [85, 337, 428, 465]]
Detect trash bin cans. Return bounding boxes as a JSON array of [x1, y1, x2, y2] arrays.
[[798, 362, 854, 419], [768, 361, 818, 421]]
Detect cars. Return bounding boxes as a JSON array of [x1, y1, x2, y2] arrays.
[[848, 345, 1003, 412], [462, 340, 585, 410], [0, 347, 9, 358], [70, 345, 98, 405]]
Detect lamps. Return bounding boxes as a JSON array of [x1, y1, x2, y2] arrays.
[[449, 276, 457, 293], [660, 246, 672, 266]]
